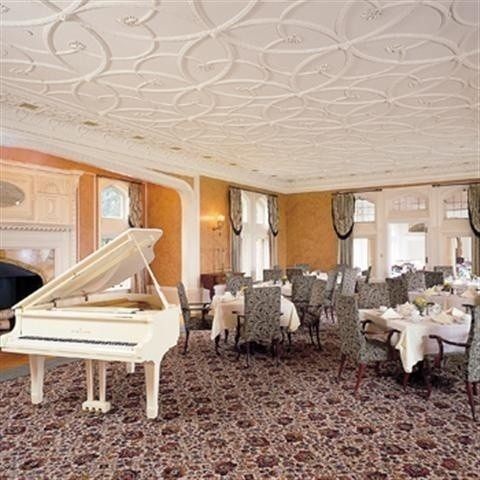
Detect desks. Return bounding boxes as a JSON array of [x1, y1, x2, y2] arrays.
[[359, 306, 473, 390]]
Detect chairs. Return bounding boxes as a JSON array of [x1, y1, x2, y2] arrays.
[[335, 295, 409, 392], [200, 264, 327, 352], [421, 304, 479, 418], [321, 262, 480, 323], [237, 287, 282, 368], [178, 282, 222, 356]]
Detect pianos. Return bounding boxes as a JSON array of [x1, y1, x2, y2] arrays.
[[0, 228, 182, 420]]
